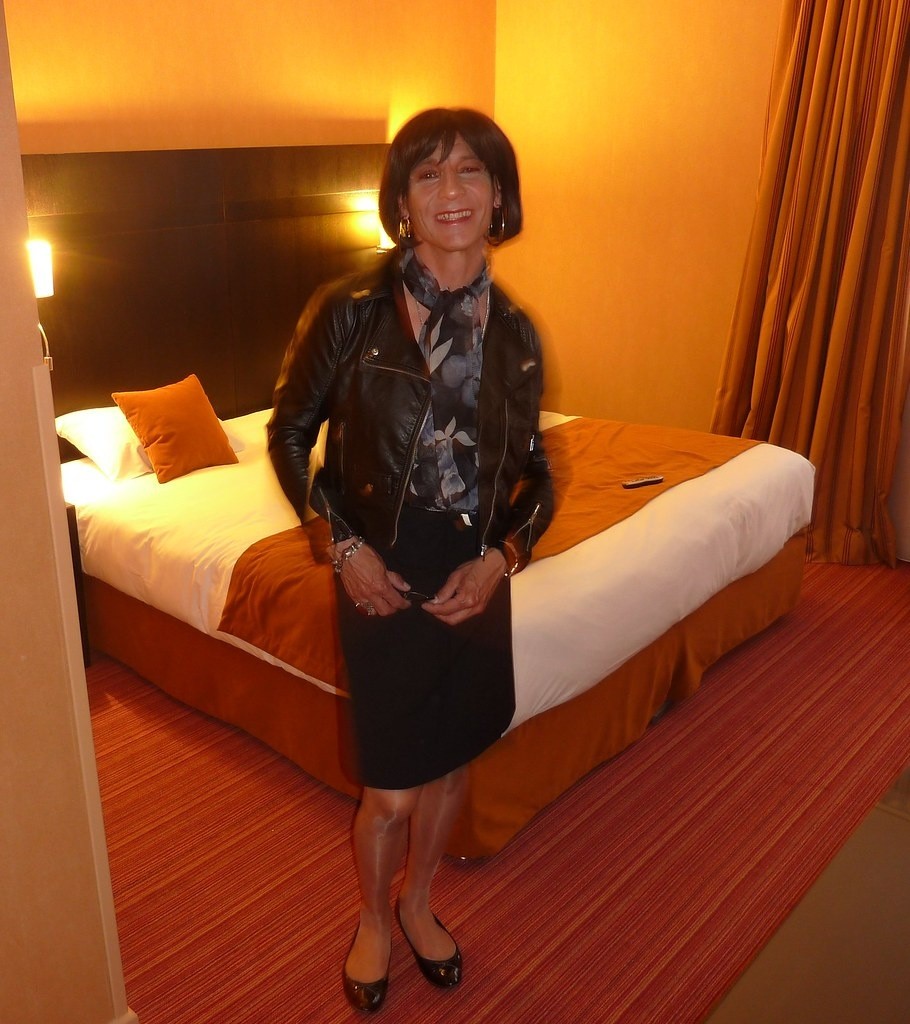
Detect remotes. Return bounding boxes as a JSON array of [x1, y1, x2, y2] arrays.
[[621, 475, 663, 488]]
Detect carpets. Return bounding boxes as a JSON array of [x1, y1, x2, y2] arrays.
[[88, 557, 910, 1024]]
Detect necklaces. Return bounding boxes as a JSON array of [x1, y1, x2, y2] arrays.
[[415, 285, 490, 341]]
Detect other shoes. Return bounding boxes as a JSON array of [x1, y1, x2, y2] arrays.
[[399, 910, 462, 989], [345, 971, 388, 1014]]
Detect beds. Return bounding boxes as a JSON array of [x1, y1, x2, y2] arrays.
[[62, 408, 819, 866]]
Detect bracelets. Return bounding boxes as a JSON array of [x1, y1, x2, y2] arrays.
[[333, 535, 365, 576]]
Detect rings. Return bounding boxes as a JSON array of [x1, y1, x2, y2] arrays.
[[355, 602, 374, 616]]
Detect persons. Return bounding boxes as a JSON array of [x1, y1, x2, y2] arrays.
[[267, 105, 557, 1016]]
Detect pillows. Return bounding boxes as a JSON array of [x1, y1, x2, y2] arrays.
[[112, 374, 241, 484], [54, 406, 243, 483]]
